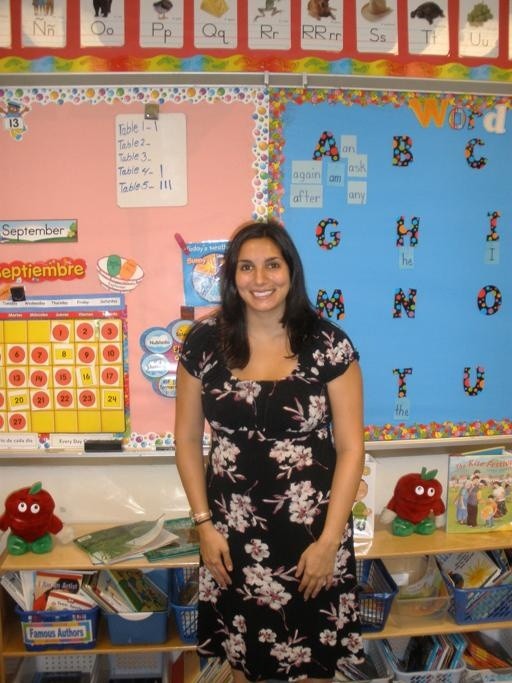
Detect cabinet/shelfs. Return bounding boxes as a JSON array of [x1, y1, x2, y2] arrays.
[[1, 512, 512, 683]]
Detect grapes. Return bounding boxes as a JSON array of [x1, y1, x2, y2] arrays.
[[466, 3, 494, 27]]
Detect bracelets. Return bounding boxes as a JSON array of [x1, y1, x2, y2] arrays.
[[190, 510, 214, 525]]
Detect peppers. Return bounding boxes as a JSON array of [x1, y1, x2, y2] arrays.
[[387, 473, 445, 523], [0, 487, 62, 543]]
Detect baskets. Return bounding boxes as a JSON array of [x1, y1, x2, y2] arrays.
[[31, 652, 97, 681], [333, 640, 396, 683], [351, 559, 400, 630], [169, 566, 207, 643], [106, 649, 163, 679], [461, 656, 511, 682]]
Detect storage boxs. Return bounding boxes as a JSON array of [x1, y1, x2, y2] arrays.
[[463, 629, 512, 682], [361, 639, 396, 683], [172, 567, 202, 643], [10, 655, 99, 683], [101, 567, 171, 645], [93, 650, 173, 683], [355, 556, 400, 634], [390, 579, 455, 629]]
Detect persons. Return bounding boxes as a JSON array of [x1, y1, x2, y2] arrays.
[[171, 221, 368, 681]]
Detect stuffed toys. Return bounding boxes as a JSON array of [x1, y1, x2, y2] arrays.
[[379, 468, 446, 535], [0, 481, 65, 556]]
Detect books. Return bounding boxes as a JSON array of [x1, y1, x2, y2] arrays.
[[353, 453, 378, 538], [446, 445, 511, 534], [0, 514, 234, 681], [333, 549, 512, 681]]
